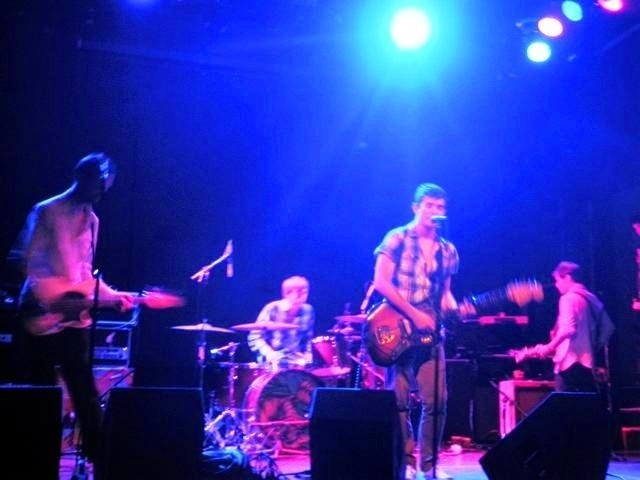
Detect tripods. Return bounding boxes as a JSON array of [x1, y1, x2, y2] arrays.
[[194, 342, 241, 449]]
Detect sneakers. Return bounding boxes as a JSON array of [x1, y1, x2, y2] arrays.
[[406, 464, 416, 480], [424, 467, 453, 480]]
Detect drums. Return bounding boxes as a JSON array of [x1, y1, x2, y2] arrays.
[[308, 335, 340, 367], [242, 368, 326, 449], [204, 362, 264, 413], [312, 366, 351, 388]]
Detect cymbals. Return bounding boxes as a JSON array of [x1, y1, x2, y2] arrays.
[[231, 321, 298, 332], [171, 323, 234, 339], [335, 314, 367, 325]]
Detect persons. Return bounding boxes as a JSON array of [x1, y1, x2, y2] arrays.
[[509, 261, 617, 394], [8, 150, 140, 465], [242, 273, 316, 420], [371, 182, 475, 479]]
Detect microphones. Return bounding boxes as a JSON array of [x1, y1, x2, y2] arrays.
[[224, 238, 234, 275], [426, 215, 448, 223]]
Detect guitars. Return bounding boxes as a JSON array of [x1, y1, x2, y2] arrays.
[[19, 278, 185, 336], [362, 278, 544, 367]]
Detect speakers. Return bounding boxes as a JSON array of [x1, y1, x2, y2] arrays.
[[94, 387, 203, 480], [479, 392, 613, 479], [308, 389, 406, 479], [0, 383, 61, 479]]
